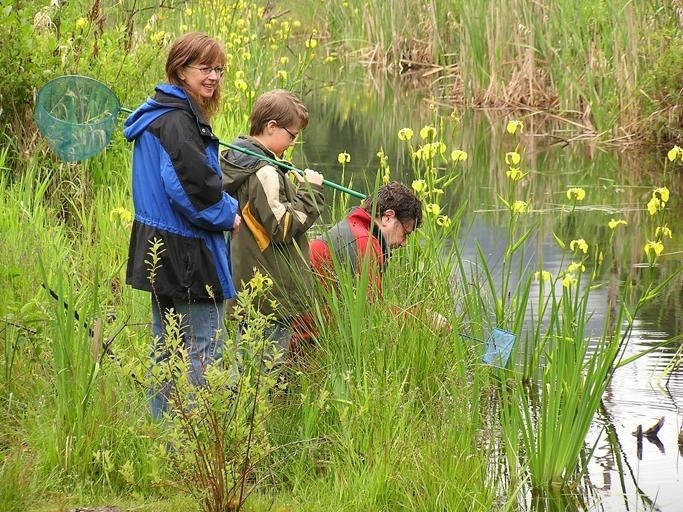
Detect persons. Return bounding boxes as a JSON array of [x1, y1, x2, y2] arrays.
[[115, 31, 243, 461], [282, 178, 455, 374], [216, 86, 327, 402]]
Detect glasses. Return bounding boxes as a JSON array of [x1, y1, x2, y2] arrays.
[[281, 127, 296, 141], [186, 64, 225, 75], [399, 220, 412, 241]]
